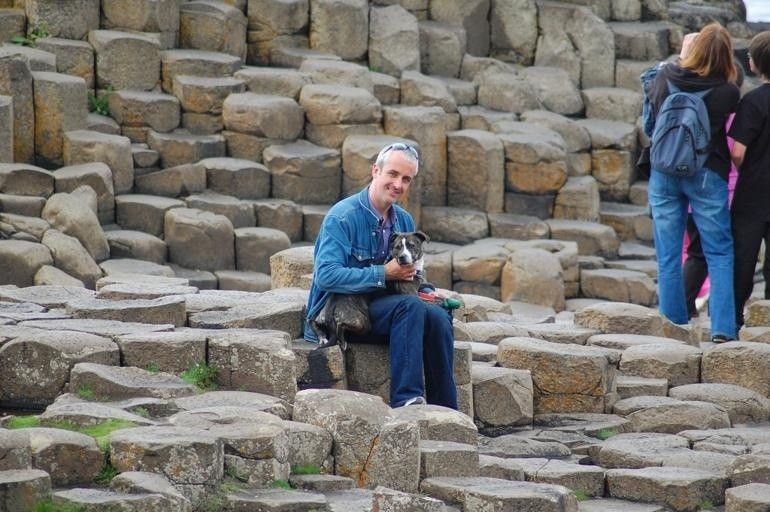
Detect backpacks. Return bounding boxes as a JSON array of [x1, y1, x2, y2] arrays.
[[650, 77, 713, 180]]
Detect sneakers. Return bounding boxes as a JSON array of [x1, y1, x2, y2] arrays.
[[713, 334, 735, 343], [402, 396, 426, 406]]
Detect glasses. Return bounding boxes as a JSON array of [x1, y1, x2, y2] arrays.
[[377, 143, 418, 167]]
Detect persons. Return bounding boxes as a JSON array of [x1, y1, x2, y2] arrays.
[[729, 27, 770, 332], [675, 52, 747, 318], [640, 20, 737, 346], [301, 141, 458, 413]]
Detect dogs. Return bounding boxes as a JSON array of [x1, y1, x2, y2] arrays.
[[309, 230, 442, 350]]
